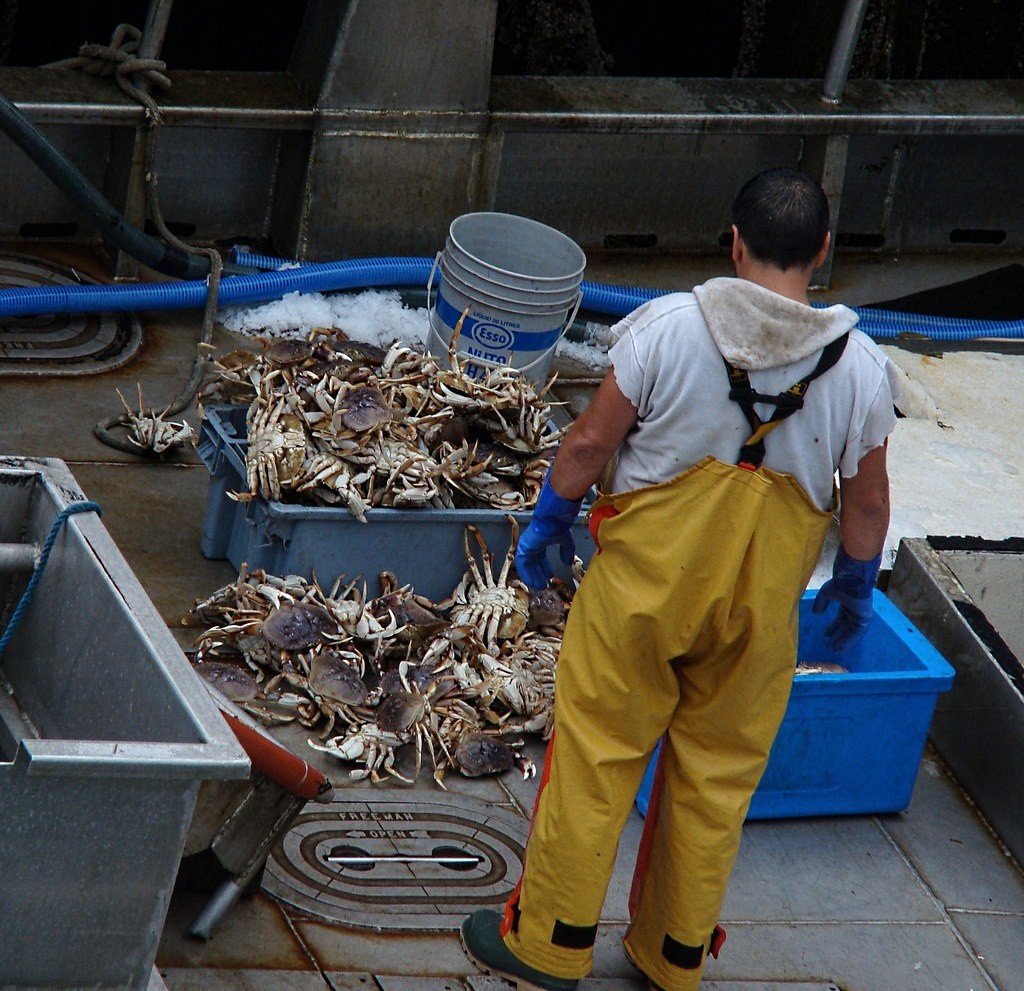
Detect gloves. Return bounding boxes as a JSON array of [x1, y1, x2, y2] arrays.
[[515, 468, 585, 590], [811, 540, 883, 653]]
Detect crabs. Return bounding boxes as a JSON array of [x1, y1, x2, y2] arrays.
[[110, 307, 854, 794]]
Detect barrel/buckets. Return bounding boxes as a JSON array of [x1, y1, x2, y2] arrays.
[[426, 212, 587, 401]]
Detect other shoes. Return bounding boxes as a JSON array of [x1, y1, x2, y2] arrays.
[[459, 908, 579, 991]]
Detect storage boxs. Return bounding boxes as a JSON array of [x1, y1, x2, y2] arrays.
[[205, 395, 600, 613], [634, 583, 955, 822], [0, 453, 251, 991]]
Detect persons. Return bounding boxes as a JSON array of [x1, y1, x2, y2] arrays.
[[460, 170, 896, 990]]
